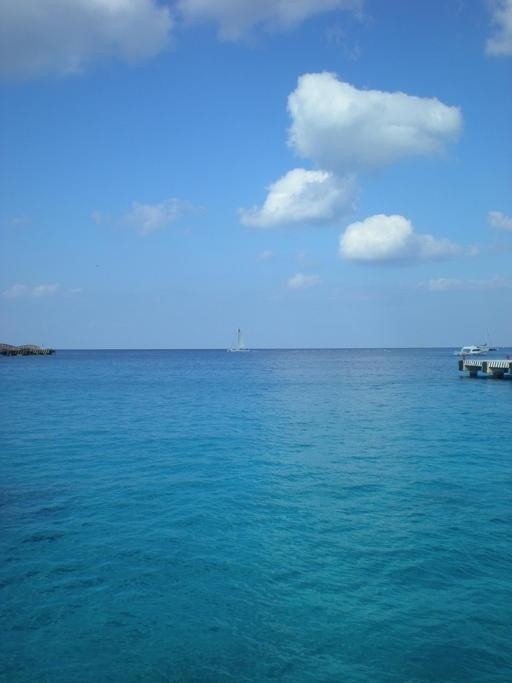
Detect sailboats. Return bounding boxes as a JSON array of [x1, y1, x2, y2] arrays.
[[480, 328, 503, 349], [227, 327, 249, 352]]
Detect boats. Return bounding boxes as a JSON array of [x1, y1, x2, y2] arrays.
[[453, 345, 489, 356]]
